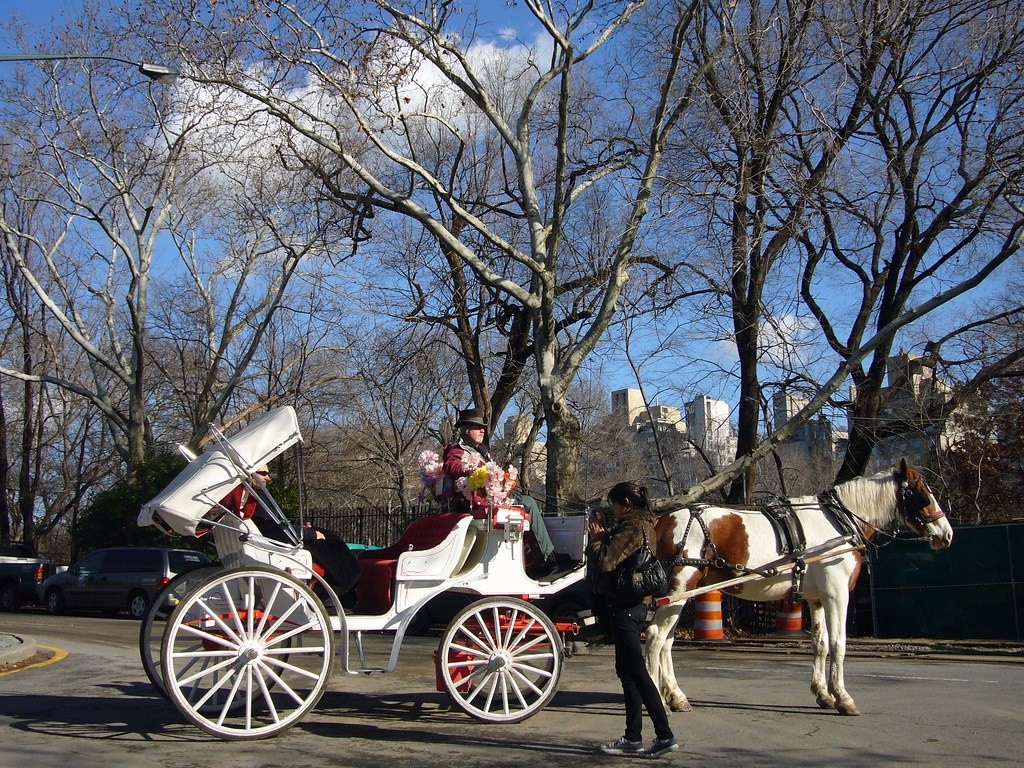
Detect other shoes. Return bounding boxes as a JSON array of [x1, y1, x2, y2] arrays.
[[535, 562, 569, 583], [341, 587, 359, 612], [532, 551, 571, 564]]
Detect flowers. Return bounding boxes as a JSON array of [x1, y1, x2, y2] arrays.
[[417, 449, 445, 503], [456, 461, 518, 508]]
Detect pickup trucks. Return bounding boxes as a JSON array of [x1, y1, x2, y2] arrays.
[[0, 540, 50, 613]]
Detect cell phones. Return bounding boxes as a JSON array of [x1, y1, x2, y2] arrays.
[[591, 509, 605, 527]]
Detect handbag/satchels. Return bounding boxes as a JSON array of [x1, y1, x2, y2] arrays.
[[592, 527, 669, 598]]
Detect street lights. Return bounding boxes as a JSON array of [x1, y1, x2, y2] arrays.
[[0, 53, 180, 85]]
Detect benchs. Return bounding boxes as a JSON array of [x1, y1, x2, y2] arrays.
[[212, 509, 324, 589], [354, 513, 473, 581], [449, 490, 531, 532]]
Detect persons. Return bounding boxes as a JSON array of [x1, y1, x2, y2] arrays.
[[218, 464, 385, 614], [583, 482, 679, 759], [442, 409, 571, 573]]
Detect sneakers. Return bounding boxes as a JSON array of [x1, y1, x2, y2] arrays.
[[599, 736, 644, 756], [639, 737, 679, 758]]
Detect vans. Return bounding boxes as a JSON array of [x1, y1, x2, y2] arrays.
[[40, 544, 216, 621]]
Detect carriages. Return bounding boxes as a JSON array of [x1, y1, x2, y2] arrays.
[[136, 403, 956, 745]]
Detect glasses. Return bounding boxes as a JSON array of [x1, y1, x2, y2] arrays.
[[471, 425, 487, 430], [256, 471, 270, 476]]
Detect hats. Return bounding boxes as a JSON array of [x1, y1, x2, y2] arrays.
[[454, 409, 489, 428]]
